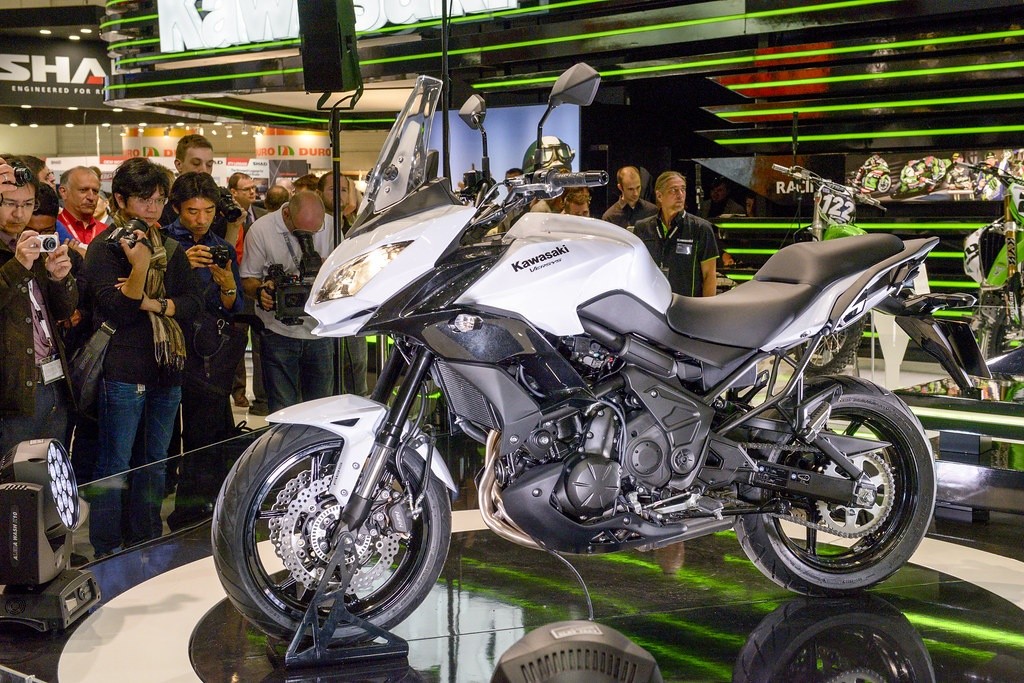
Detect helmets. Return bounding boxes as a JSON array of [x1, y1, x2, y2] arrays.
[[522, 135, 575, 184], [963, 226, 1006, 286]]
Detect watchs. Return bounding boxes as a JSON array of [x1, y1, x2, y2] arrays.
[[222, 289, 237, 296], [158, 297, 167, 316]]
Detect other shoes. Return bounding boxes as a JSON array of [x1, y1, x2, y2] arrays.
[[234, 391, 247, 407]]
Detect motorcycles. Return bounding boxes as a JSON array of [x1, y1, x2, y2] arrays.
[[845, 159, 1002, 202], [769, 162, 1024, 389], [209, 57, 997, 642]]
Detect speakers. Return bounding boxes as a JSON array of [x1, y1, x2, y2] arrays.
[[298, 0, 362, 93]]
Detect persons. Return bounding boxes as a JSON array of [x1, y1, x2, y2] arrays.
[[0, 156, 17, 193], [0, 154, 87, 586], [603, 166, 659, 228], [503, 168, 591, 217], [82, 157, 197, 558], [23, 133, 369, 530], [633, 170, 722, 297], [700, 178, 777, 291]]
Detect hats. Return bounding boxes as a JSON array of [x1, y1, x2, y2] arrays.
[[952, 152, 963, 159], [985, 152, 995, 159]]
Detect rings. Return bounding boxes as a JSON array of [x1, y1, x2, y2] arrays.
[[3, 173, 8, 182]]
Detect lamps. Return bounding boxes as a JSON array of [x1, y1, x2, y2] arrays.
[[164, 126, 171, 136], [120, 126, 125, 136], [196, 126, 265, 139], [0, 439, 98, 631], [138, 125, 144, 133], [490, 620, 662, 683]]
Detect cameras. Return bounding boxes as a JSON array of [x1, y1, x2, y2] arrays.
[[215, 187, 242, 222], [30, 235, 60, 252], [203, 245, 230, 269], [105, 216, 151, 267], [8, 162, 32, 187]]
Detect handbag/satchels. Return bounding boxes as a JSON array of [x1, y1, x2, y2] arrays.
[[181, 312, 250, 388]]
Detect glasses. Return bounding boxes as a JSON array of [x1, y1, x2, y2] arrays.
[[140, 196, 169, 206], [236, 185, 256, 191], [289, 212, 325, 232], [2, 198, 40, 211], [533, 143, 575, 167]]
